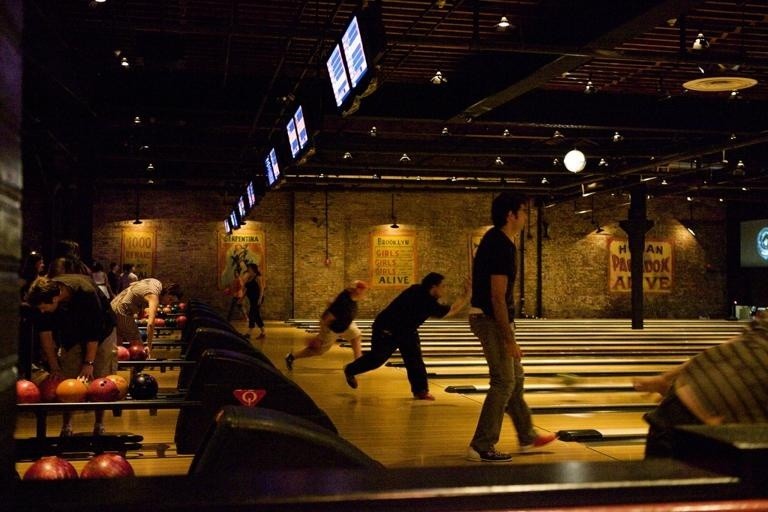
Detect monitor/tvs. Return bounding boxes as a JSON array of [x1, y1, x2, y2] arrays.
[[224, 146, 284, 234], [286, 106, 311, 160], [327, 10, 374, 115]]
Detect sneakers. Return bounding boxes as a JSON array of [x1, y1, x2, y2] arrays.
[[256, 332, 265, 338], [415, 391, 435, 399], [466, 445, 513, 462], [243, 332, 251, 339], [285, 352, 294, 368], [518, 433, 560, 453], [343, 365, 358, 388]]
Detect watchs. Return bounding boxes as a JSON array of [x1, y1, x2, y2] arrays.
[[84, 358, 96, 366]]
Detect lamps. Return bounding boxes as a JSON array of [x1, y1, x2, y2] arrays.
[[119, 57, 154, 185]]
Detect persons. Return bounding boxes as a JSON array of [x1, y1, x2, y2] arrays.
[[464, 190, 564, 462], [22, 272, 119, 453], [47, 239, 93, 277], [18, 252, 46, 382], [631, 307, 768, 458], [245, 265, 268, 340], [224, 267, 249, 324], [343, 272, 472, 401], [93, 260, 142, 300], [110, 278, 182, 360], [285, 280, 377, 371]]
